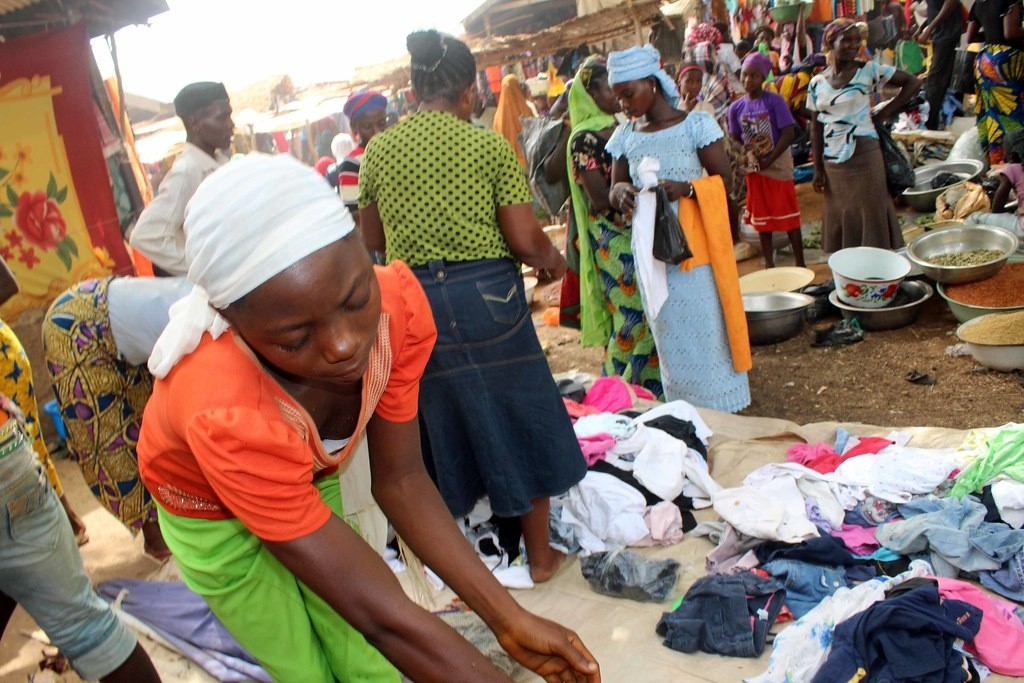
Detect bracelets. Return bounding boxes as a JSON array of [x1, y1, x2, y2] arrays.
[[686, 180, 693, 198]]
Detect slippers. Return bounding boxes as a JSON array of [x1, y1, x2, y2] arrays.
[[144, 550, 170, 565]]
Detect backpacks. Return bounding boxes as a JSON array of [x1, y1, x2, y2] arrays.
[[895, 37, 923, 74]]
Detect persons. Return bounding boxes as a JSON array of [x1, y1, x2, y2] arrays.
[[676, 0, 1024, 253], [136, 150, 602, 683], [566, 53, 665, 402], [728, 52, 807, 269], [604, 44, 753, 413], [674, 61, 715, 177], [355, 29, 589, 583], [805, 17, 920, 251], [0, 254, 89, 545], [0, 319, 164, 683], [129, 82, 235, 277], [263, 73, 580, 329], [42, 274, 196, 558]]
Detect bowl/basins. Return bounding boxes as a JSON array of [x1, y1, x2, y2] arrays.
[[827, 279, 934, 332], [766, 2, 814, 23], [828, 245, 912, 308], [936, 281, 1024, 325], [738, 267, 815, 344], [523, 276, 538, 305], [906, 224, 1020, 284], [956, 312, 1024, 373], [901, 159, 985, 213]]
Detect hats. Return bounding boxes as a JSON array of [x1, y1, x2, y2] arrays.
[[741, 52, 773, 79], [343, 91, 387, 120]]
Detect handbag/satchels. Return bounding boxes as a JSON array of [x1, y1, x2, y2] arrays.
[[871, 119, 915, 198], [653, 186, 693, 265]]
